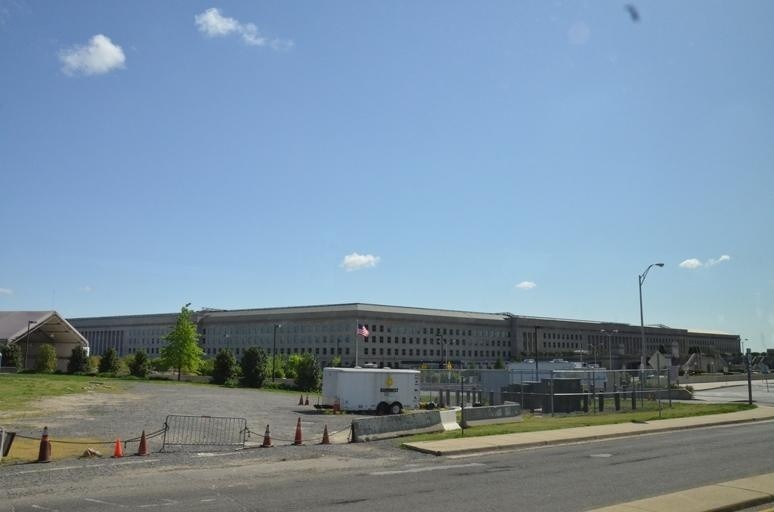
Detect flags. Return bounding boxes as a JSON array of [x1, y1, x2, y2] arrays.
[[356, 322, 369, 337]]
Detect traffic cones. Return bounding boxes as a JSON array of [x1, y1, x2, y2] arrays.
[[319, 424, 332, 445], [260, 423, 274, 448], [134, 430, 150, 456], [290, 417, 306, 446], [110, 437, 124, 458], [33, 426, 51, 463], [298, 392, 309, 405]]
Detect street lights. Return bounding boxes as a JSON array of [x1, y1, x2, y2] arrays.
[[600, 328, 619, 370], [438, 338, 457, 364], [23, 320, 37, 369], [589, 342, 604, 368], [638, 262, 664, 384], [272, 324, 282, 382]]
